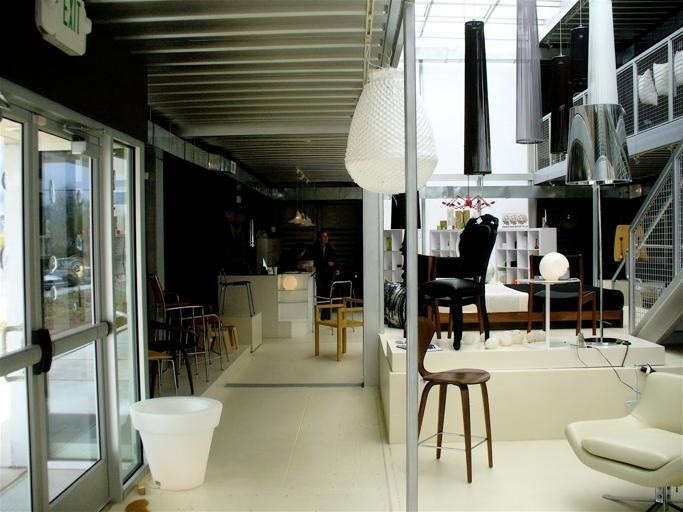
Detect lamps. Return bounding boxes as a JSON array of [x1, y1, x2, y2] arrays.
[[462, 2, 619, 176], [564, 101, 632, 348], [568, 0, 590, 41], [551, 0, 569, 66], [289, 168, 315, 228], [342, 41, 438, 197]]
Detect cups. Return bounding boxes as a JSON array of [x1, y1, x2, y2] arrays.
[[267, 266, 277, 275]]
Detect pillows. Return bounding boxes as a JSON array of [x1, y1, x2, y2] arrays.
[[633, 69, 661, 109], [649, 61, 679, 101], [672, 49, 683, 86]]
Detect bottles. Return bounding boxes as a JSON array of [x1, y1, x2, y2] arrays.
[[385, 236, 391, 251]]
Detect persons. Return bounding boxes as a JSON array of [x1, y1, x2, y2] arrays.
[[302, 229, 341, 321]]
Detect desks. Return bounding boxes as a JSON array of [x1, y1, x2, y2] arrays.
[[517, 276, 581, 340], [596, 278, 666, 331]]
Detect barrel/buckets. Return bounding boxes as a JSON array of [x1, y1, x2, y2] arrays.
[[128, 396, 223, 492]]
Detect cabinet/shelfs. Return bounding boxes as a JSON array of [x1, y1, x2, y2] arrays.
[[383, 227, 425, 283], [496, 227, 557, 286], [276, 273, 308, 322], [428, 228, 496, 284]]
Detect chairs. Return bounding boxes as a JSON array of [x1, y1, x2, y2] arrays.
[[418, 212, 501, 351], [404, 315, 496, 484], [139, 269, 265, 395], [312, 296, 363, 364], [313, 280, 357, 335], [565, 367, 683, 512], [527, 251, 597, 340]]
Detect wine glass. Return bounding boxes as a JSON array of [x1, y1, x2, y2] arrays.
[[502, 215, 528, 230]]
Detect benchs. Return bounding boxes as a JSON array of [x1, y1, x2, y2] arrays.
[[430, 280, 625, 329]]
